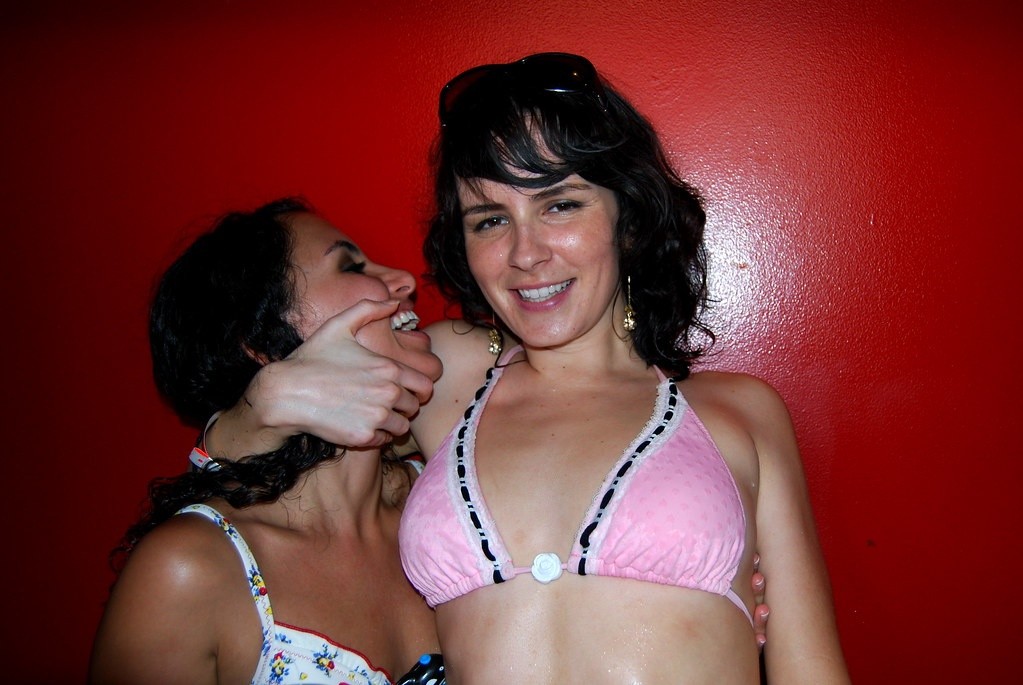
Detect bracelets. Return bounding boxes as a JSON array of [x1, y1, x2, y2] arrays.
[[188, 409, 224, 470]]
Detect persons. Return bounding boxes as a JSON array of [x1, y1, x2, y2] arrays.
[[87, 194, 447, 685], [188, 52, 852, 685]]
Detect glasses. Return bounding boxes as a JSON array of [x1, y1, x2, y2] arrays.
[[439, 52, 614, 134]]
